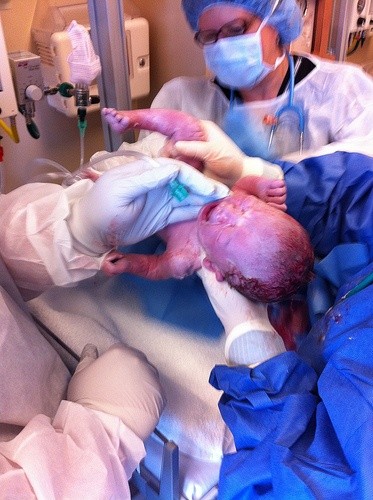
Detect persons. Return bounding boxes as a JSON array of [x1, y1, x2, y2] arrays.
[[0, 157, 233, 500], [84, 103, 373, 500], [137, 0, 373, 163]]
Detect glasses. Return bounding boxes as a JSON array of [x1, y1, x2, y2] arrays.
[[192, 19, 249, 46]]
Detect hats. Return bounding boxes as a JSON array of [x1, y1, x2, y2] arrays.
[[182, 0, 302, 44]]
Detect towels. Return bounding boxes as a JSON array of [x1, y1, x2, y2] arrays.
[[24, 277, 238, 462]]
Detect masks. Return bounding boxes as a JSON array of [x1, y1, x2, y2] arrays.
[[202, 33, 272, 89]]
